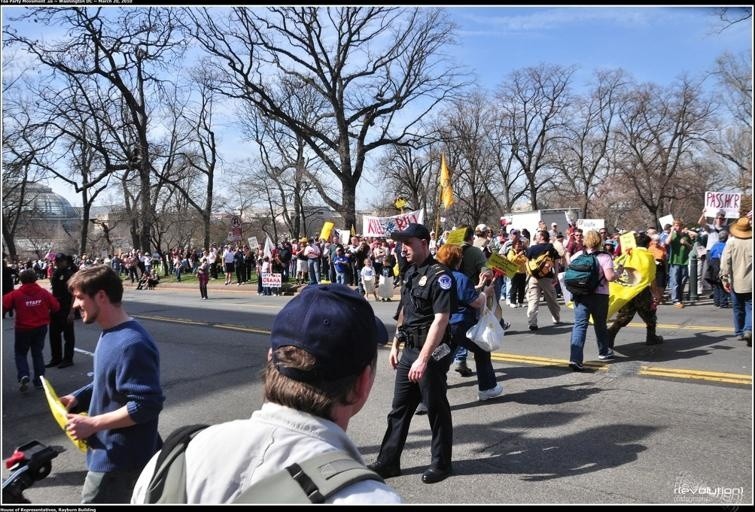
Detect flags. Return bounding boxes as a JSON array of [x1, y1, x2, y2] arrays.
[[439, 151, 455, 211]]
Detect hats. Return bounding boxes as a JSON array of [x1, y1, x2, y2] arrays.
[[55, 253, 65, 261], [271, 283, 388, 382], [476, 224, 488, 232], [603, 239, 615, 245], [551, 223, 557, 226], [730, 218, 752, 239], [391, 224, 430, 241]]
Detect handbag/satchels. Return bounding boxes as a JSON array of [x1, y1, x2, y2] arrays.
[[466, 310, 504, 353], [526, 248, 555, 280]]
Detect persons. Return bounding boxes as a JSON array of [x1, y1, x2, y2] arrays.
[[2, 206, 753, 416], [364, 224, 459, 484], [131, 280, 404, 505], [55, 264, 164, 506]]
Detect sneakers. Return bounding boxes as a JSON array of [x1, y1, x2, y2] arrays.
[[674, 303, 684, 308], [598, 351, 615, 362], [744, 332, 752, 346], [415, 403, 428, 415], [529, 328, 536, 332], [510, 303, 517, 308], [737, 335, 743, 340], [647, 336, 663, 345], [477, 384, 503, 401], [33, 383, 44, 391], [518, 303, 527, 308], [455, 366, 473, 377], [503, 321, 510, 330], [569, 360, 584, 371], [18, 376, 30, 393]]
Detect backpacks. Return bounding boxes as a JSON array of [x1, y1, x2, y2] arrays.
[[564, 250, 607, 296]]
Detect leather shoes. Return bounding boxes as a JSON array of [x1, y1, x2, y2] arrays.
[[44, 361, 58, 368], [57, 360, 74, 368], [367, 461, 400, 477], [422, 463, 452, 483]]
